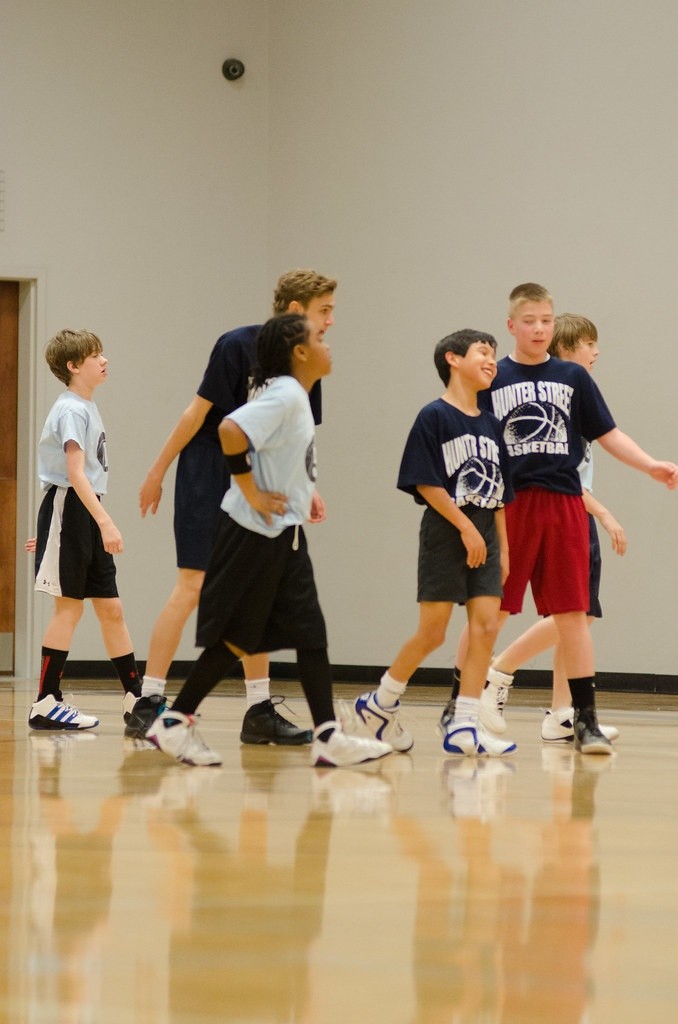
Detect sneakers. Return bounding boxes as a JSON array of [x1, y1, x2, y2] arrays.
[[241, 694, 314, 747], [573, 708, 613, 754], [121, 692, 141, 723], [438, 700, 456, 734], [123, 738, 156, 757], [123, 694, 171, 735], [442, 719, 518, 758], [310, 718, 393, 768], [541, 707, 620, 740], [144, 710, 222, 766], [352, 691, 414, 753], [29, 693, 98, 731], [479, 678, 515, 734]]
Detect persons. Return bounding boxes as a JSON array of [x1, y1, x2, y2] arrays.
[[354, 328, 517, 757], [25, 329, 200, 730], [437, 282, 678, 755], [143, 312, 392, 768], [478, 312, 627, 745], [124, 269, 337, 745]]
